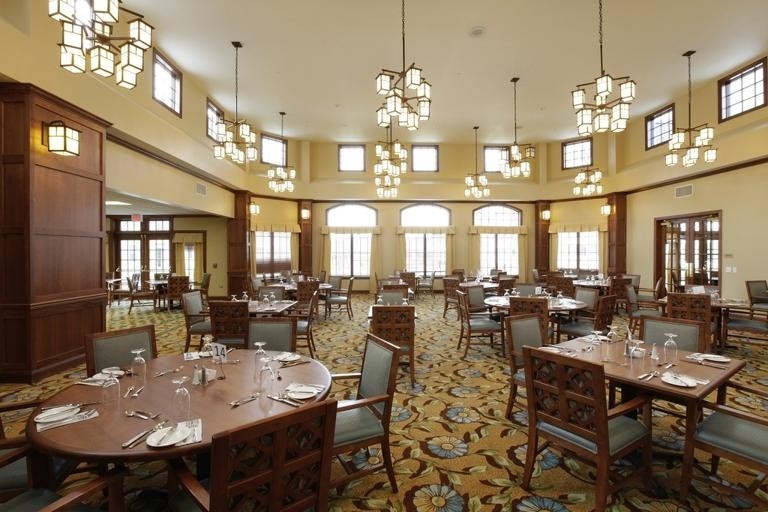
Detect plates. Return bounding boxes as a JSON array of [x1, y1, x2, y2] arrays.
[[34, 349, 317, 448], [537, 335, 731, 388]]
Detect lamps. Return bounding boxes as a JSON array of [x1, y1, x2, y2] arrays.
[[213, 42, 258, 163], [300, 207, 310, 220], [665, 51, 717, 169], [600, 205, 611, 215], [248, 200, 259, 216], [45, 0, 154, 90], [371, 131, 409, 199], [463, 127, 494, 198], [571, 170, 602, 197], [376, 2, 429, 129], [264, 114, 297, 192], [40, 119, 81, 158], [570, 1, 636, 139], [501, 78, 533, 180], [540, 210, 551, 220]]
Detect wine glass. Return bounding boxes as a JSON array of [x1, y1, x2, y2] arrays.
[[160, 274, 170, 282], [686, 290, 720, 305], [279, 277, 320, 289], [463, 275, 493, 285], [377, 296, 409, 305], [504, 287, 564, 303], [563, 269, 616, 286], [101, 335, 275, 424], [231, 290, 276, 309], [590, 326, 709, 383]]
